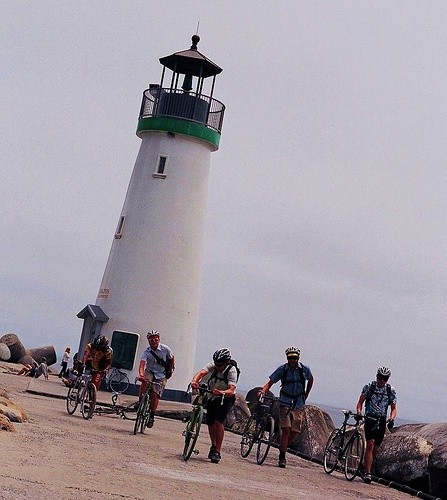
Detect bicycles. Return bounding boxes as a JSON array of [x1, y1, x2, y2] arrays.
[[182, 382, 226, 461], [90, 367, 130, 394], [80, 394, 141, 421], [66, 365, 107, 420], [240, 393, 295, 465], [324, 409, 378, 481], [133, 375, 163, 436]]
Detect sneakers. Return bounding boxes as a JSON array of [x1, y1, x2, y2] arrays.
[[365, 474, 372, 484], [147, 418, 154, 428], [211, 452, 222, 464], [134, 402, 140, 409], [208, 446, 217, 459], [279, 460, 286, 468], [362, 473, 367, 480]]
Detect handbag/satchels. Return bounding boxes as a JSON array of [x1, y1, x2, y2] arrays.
[[146, 348, 175, 380]]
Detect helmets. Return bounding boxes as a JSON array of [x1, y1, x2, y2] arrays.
[[377, 367, 391, 379], [94, 334, 109, 347], [213, 349, 231, 363], [146, 330, 160, 339]]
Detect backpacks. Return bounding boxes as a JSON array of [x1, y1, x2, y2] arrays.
[[208, 360, 240, 390]]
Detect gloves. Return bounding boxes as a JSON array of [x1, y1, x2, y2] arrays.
[[353, 413, 363, 421], [386, 418, 394, 429]]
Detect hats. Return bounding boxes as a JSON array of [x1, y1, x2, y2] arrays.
[[285, 347, 301, 357]]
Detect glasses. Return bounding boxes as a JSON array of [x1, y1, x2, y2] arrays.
[[148, 339, 159, 343], [214, 364, 223, 368], [379, 378, 387, 382], [286, 356, 299, 361]]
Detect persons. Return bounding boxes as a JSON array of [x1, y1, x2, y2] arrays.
[[17, 357, 49, 380], [357, 367, 398, 483], [256, 347, 314, 468], [191, 348, 237, 464], [133, 330, 175, 428], [81, 334, 113, 392], [58, 348, 71, 378], [73, 353, 78, 369], [24, 362, 38, 379]]
